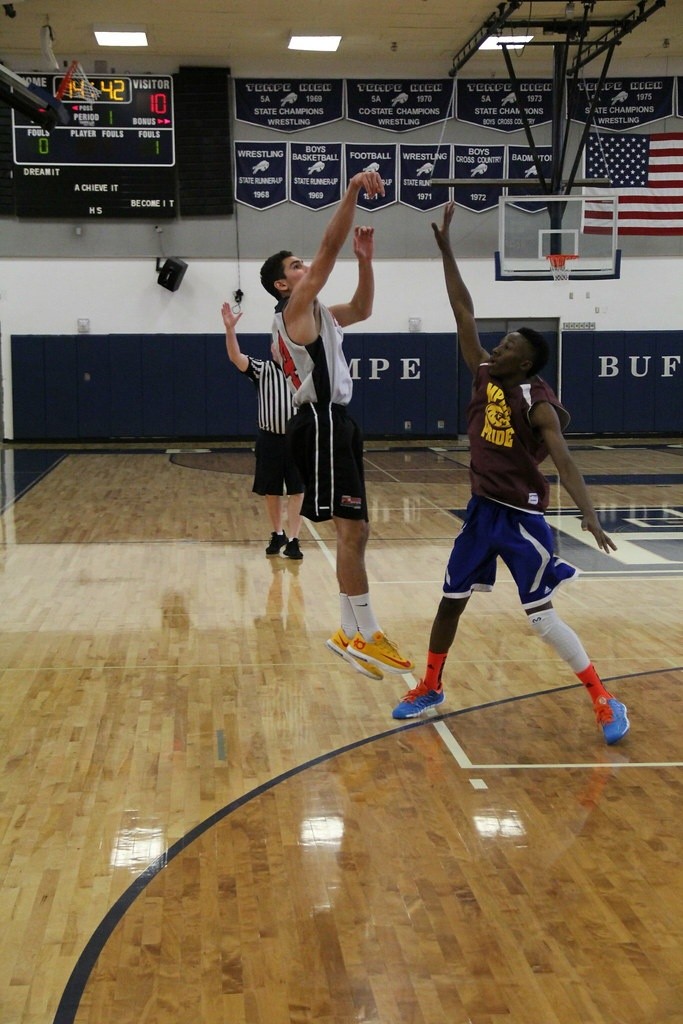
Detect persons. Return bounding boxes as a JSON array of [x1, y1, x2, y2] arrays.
[[391, 206, 630, 746], [220, 303, 308, 560], [259, 171, 416, 680]]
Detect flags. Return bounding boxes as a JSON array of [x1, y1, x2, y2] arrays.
[[582, 132, 682, 239]]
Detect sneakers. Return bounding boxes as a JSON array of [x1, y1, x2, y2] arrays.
[[283, 538, 303, 559], [266, 529, 289, 554], [325, 628, 384, 681], [392, 678, 445, 719], [347, 631, 416, 674], [593, 690, 630, 745]]
